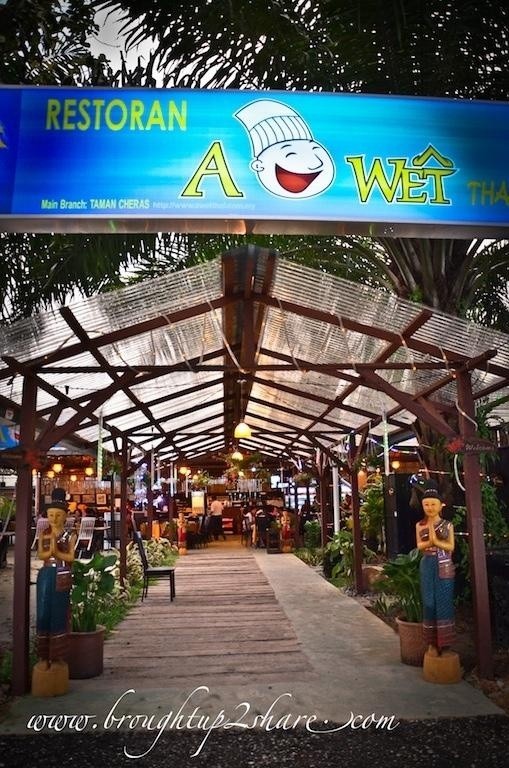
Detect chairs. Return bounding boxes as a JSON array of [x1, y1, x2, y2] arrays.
[[237, 513, 253, 547], [129, 516, 176, 603], [30, 516, 96, 560], [187, 514, 211, 549]]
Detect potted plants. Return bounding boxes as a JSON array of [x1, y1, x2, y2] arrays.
[[378, 546, 440, 667], [60, 553, 118, 681], [294, 472, 311, 487]]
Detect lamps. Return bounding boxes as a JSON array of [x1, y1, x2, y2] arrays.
[[234, 379, 252, 439]]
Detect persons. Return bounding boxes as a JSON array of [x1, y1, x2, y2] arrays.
[[208, 495, 225, 542], [236, 495, 320, 545], [71, 503, 118, 530], [415, 479, 457, 657], [34, 488, 77, 672], [339, 494, 352, 516]]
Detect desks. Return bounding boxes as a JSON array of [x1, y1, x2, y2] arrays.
[[31, 526, 112, 555]]
